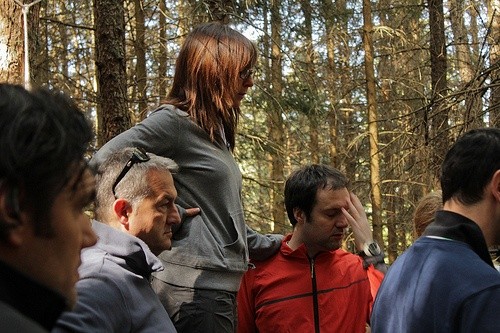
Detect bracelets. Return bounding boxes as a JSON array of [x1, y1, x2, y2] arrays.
[[361, 256, 384, 271]]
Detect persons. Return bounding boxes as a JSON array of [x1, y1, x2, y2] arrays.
[[85, 19, 288, 333], [50, 147, 181, 333], [411, 190, 500, 273], [370, 127, 500, 333], [234, 161, 391, 333], [0, 79, 103, 333]]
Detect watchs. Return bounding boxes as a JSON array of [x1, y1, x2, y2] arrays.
[[357, 239, 382, 260]]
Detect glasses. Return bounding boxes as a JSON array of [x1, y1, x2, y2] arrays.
[[67, 154, 102, 200], [239, 68, 256, 80], [112, 145, 151, 200]]
